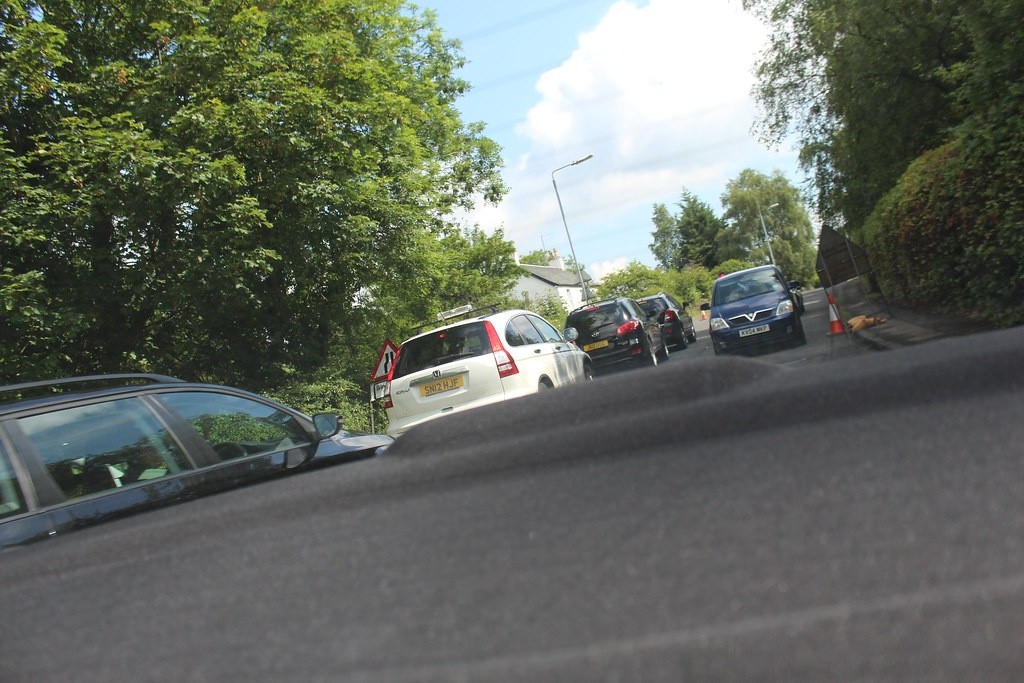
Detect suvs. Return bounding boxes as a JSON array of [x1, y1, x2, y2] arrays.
[[0, 371, 400, 558], [565, 298, 668, 373], [387, 303, 594, 444], [635, 290, 697, 349]]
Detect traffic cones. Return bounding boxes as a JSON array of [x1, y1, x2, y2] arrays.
[[826, 290, 851, 335]]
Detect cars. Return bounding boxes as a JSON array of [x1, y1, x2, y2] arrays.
[[699, 264, 807, 353]]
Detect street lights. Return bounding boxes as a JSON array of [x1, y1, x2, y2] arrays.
[[758, 203, 781, 269], [550, 154, 592, 310]]
